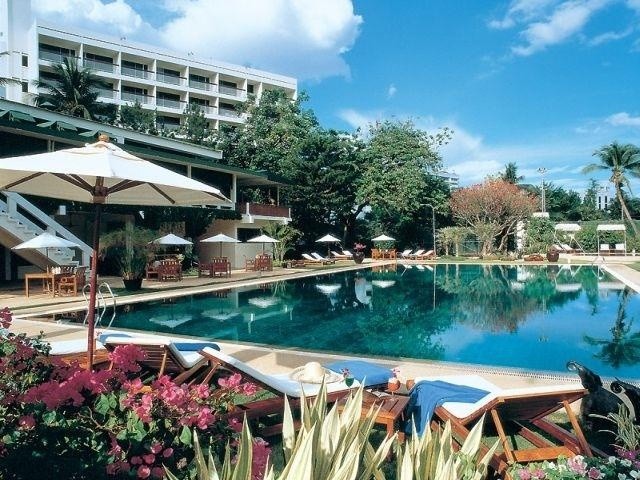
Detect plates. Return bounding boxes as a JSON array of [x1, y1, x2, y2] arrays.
[[287, 365, 345, 384]]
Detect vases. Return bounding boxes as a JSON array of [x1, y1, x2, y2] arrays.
[[547, 251, 559, 261]]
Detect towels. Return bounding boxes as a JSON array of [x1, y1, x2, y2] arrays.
[[405, 380, 491, 437], [322, 359, 396, 386], [99, 332, 130, 342], [174, 343, 220, 351]]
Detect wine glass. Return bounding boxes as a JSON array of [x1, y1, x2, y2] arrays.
[[345, 374, 356, 399], [387, 377, 401, 402]]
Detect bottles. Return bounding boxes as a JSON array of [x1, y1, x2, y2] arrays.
[[50, 266, 78, 275]]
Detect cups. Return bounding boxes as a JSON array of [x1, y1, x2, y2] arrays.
[[405, 379, 417, 393], [303, 362, 324, 378]]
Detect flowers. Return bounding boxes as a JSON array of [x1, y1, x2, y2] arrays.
[[354, 243, 367, 250]]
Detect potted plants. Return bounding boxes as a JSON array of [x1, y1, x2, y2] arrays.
[[99, 227, 159, 291]]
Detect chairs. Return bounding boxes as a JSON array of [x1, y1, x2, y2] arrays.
[[301, 249, 436, 266], [145, 254, 273, 282], [25, 266, 87, 298], [96, 332, 220, 385], [406, 374, 593, 480], [172, 348, 396, 445], [550, 243, 626, 257]]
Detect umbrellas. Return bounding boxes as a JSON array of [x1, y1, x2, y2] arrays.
[[247, 235, 281, 270], [149, 233, 195, 279], [372, 265, 397, 289], [317, 275, 342, 296], [200, 290, 240, 323], [371, 234, 396, 261], [149, 297, 193, 330], [315, 234, 341, 258], [200, 232, 242, 277], [1, 135, 233, 375], [11, 232, 80, 291], [248, 285, 280, 309]]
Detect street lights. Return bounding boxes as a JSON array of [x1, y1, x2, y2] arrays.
[[420, 264, 437, 317], [536, 166, 549, 211], [420, 202, 437, 260], [535, 266, 549, 346]]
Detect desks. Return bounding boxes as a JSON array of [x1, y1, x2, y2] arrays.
[[61, 350, 112, 369], [334, 389, 410, 463]]
